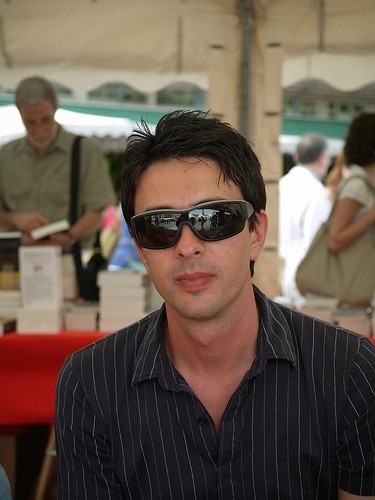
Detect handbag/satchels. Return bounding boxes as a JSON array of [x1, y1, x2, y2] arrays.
[[296, 175, 374, 307], [75, 254, 108, 303]]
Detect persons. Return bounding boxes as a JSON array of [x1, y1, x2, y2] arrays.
[[53, 109, 375, 499], [0, 77, 121, 301], [274, 134, 343, 297], [327, 112, 375, 342]]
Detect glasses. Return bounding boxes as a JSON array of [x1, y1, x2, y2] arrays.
[[129, 201, 255, 250]]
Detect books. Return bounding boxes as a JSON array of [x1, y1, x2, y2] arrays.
[[1, 219, 153, 335], [271, 294, 371, 339]]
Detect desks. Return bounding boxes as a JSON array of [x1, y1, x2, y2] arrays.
[[0, 329, 110, 500]]
